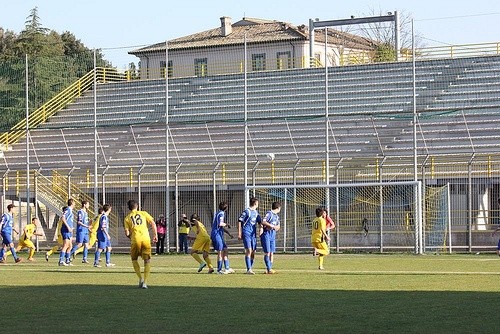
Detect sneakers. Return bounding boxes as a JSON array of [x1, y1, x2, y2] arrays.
[[208, 268, 214, 273], [198, 262, 206, 272]]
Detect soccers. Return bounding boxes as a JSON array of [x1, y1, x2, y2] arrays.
[[266, 153, 275, 161]]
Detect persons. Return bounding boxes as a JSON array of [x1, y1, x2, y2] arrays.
[[45, 206, 68, 261], [322, 209, 335, 244], [238, 198, 264, 274], [210, 202, 235, 274], [73, 207, 104, 262], [58, 199, 75, 266], [259, 202, 281, 273], [154, 213, 167, 255], [0, 204, 23, 263], [311, 208, 331, 270], [188, 213, 214, 273], [3, 218, 43, 261], [178, 214, 191, 255], [497, 239, 500, 256], [93, 204, 116, 268], [65, 200, 93, 264], [124, 199, 158, 289]]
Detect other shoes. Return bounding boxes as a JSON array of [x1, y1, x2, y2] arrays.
[[142, 284, 148, 289], [28, 257, 35, 261], [67, 262, 73, 266], [82, 260, 89, 263], [218, 269, 228, 275], [106, 263, 115, 266], [94, 264, 101, 268], [73, 251, 75, 259], [65, 255, 72, 262], [154, 253, 158, 255], [225, 268, 235, 273], [44, 252, 49, 261], [59, 262, 68, 266], [247, 270, 255, 275], [313, 247, 318, 256], [264, 269, 276, 274], [139, 280, 143, 287], [319, 266, 325, 270], [15, 258, 21, 263]]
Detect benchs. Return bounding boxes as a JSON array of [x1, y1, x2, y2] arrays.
[[0, 53, 500, 190]]
